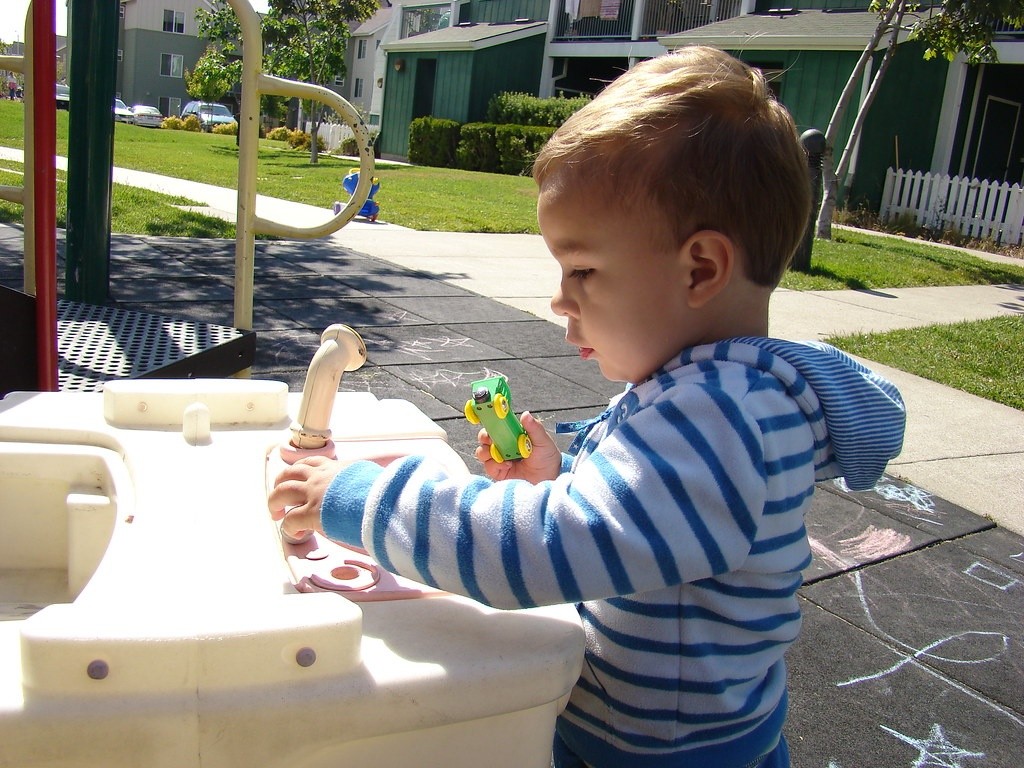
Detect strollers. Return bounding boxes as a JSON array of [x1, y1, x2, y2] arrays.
[[333, 168, 381, 222]]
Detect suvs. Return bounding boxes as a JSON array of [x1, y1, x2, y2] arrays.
[[114, 99, 134, 125], [55, 83, 70, 112]]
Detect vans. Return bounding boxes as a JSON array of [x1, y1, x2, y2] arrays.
[[180, 101, 237, 128]]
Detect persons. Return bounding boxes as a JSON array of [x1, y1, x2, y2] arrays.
[[8, 71, 17, 101], [269, 45, 912, 768]]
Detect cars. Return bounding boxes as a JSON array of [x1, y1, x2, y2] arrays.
[[131, 104, 163, 128]]
